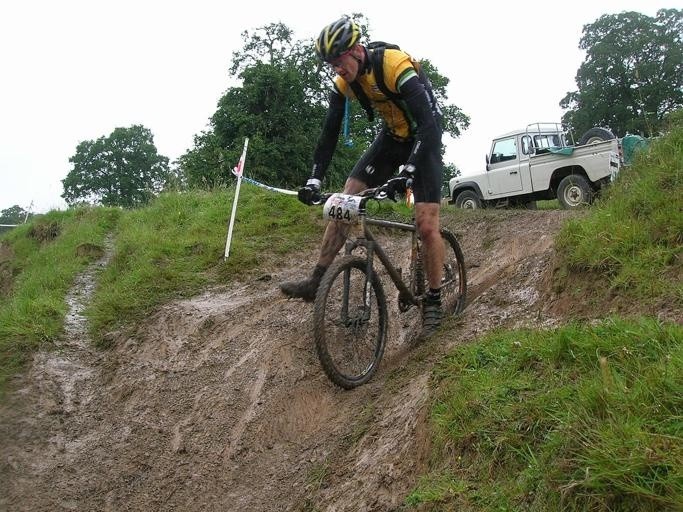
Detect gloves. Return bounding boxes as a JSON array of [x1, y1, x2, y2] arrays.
[[298, 178, 323, 206], [386, 163, 418, 202]]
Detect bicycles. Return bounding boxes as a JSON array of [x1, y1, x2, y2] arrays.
[[300, 138, 468, 390]]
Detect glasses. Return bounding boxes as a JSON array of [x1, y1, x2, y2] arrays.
[[328, 47, 353, 65]]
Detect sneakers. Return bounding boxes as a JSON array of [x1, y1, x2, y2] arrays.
[[279, 278, 320, 302], [424, 305, 443, 333]]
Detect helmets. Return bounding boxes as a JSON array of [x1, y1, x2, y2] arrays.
[[316, 17, 361, 61]]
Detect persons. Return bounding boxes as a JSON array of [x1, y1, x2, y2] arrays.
[[280, 16, 446, 340]]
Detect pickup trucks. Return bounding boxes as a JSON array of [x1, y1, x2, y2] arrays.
[[444, 120, 657, 212]]
